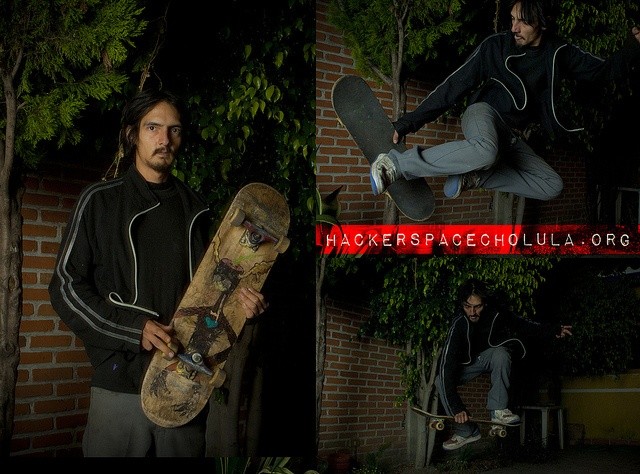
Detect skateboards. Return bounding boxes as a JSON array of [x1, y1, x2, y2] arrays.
[[141, 183, 290, 428], [412, 407, 520, 436], [331, 73, 436, 220]]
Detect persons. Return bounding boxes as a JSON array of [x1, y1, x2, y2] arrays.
[[434, 278, 574, 450], [368, 1, 640, 200], [48, 87, 271, 459]]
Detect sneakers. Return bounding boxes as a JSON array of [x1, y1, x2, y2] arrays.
[[491, 409, 520, 424], [370, 153, 398, 196], [442, 427, 482, 450], [443, 174, 481, 199]]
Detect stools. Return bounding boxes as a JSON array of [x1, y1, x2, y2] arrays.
[[516, 404, 565, 451]]
